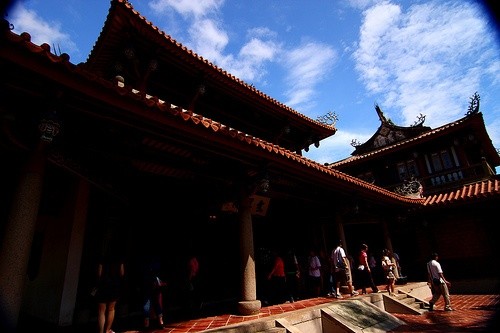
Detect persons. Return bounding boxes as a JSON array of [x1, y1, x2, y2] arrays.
[[142, 247, 199, 333], [262, 249, 294, 306], [294, 239, 407, 298], [96, 237, 125, 333], [426, 253, 452, 311]]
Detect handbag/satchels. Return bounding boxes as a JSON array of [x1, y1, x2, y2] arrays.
[[381, 257, 392, 272], [335, 260, 346, 272], [295, 264, 302, 281], [144, 299, 151, 311], [86, 282, 99, 296], [358, 263, 365, 271], [432, 278, 441, 287]]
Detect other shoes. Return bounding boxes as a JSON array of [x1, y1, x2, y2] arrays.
[[444, 307, 452, 311], [336, 294, 343, 298], [106, 329, 115, 333], [377, 290, 381, 293], [351, 293, 358, 297], [429, 300, 433, 309], [362, 292, 368, 295]]
